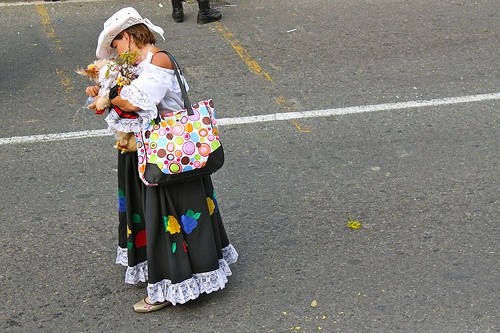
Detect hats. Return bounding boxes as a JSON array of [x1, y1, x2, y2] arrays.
[[96, 7, 165, 59]]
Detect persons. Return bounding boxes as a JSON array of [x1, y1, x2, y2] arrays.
[[170, 0, 223, 23], [84, 6, 239, 312]]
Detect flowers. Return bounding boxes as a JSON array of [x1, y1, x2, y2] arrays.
[[120, 51, 140, 78]]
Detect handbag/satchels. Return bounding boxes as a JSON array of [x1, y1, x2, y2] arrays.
[[134, 51, 224, 187]]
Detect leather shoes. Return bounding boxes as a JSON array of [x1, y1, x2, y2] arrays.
[[133, 299, 170, 313]]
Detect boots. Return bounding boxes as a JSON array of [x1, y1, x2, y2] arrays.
[[196, 0, 222, 25], [172, 0, 184, 23]]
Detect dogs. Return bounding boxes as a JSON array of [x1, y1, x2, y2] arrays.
[[85, 59, 142, 155]]
